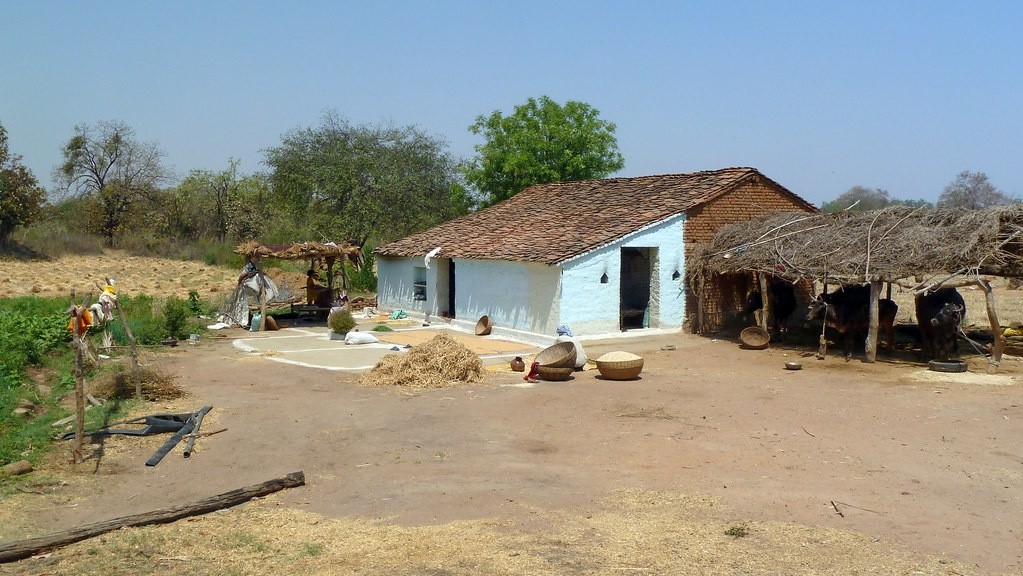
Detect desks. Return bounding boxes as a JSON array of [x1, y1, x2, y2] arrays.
[[291, 304, 323, 325]]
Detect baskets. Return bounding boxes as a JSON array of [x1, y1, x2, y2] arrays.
[[534, 366, 576, 381], [594, 356, 645, 380], [266, 315, 280, 331], [739, 326, 771, 350], [533, 341, 578, 368], [474, 314, 491, 336]]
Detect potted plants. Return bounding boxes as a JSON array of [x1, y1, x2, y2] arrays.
[[327, 306, 358, 340]]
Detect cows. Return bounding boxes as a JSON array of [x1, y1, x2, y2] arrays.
[[806, 283, 967, 364]]
